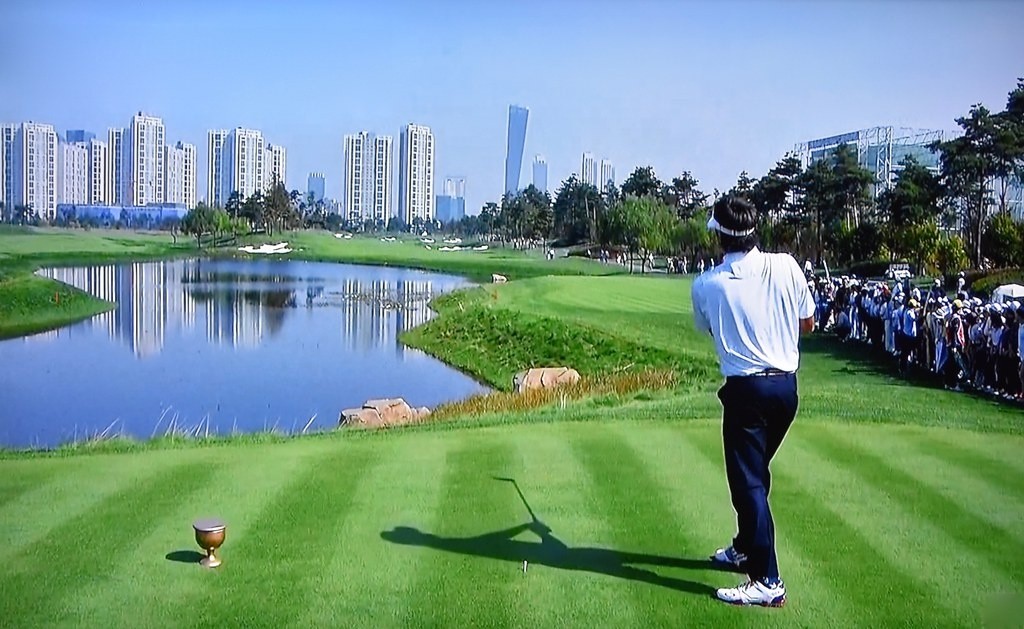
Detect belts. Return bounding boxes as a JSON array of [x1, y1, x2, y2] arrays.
[[754, 365, 790, 376]]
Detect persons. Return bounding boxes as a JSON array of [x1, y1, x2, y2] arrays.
[[547, 248, 555, 260], [697, 254, 1023, 410], [691, 196, 816, 608], [587, 247, 688, 276]]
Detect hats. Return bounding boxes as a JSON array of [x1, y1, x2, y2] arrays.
[[806, 270, 1024, 322], [707, 204, 755, 236]]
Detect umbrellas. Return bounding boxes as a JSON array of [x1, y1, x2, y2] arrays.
[[994, 283, 1024, 299]]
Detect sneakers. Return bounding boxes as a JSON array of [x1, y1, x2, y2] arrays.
[[714, 545, 749, 568], [715, 574, 786, 608]]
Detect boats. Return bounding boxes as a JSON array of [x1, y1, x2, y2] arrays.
[[240, 242, 293, 254]]
